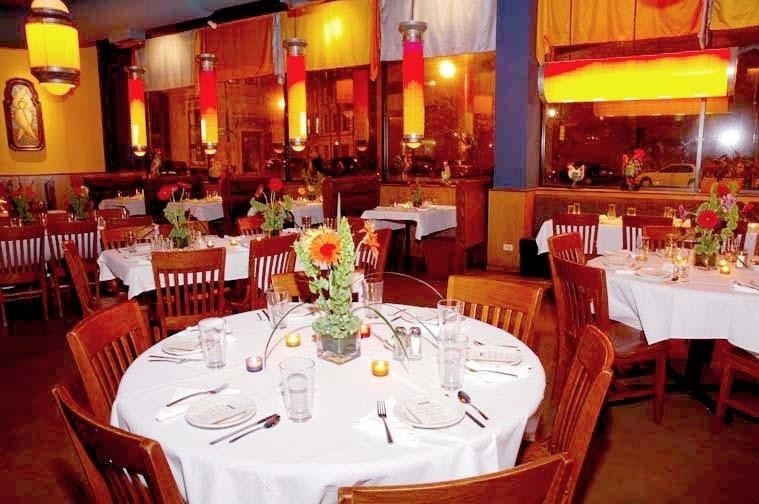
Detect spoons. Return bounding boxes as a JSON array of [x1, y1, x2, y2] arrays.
[[230, 414, 280, 443], [457, 390, 489, 421]]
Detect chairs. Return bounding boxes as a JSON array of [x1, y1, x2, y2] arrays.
[[158, 219, 209, 236], [47, 221, 100, 317], [231, 232, 300, 312], [151, 247, 231, 337], [63, 238, 149, 325], [644, 226, 687, 249], [352, 227, 392, 284], [100, 225, 156, 249], [551, 213, 600, 255], [446, 275, 544, 339], [348, 216, 367, 231], [0, 224, 48, 327], [236, 215, 264, 234], [622, 214, 672, 252], [266, 267, 367, 302], [51, 383, 182, 504], [552, 253, 667, 425], [517, 324, 615, 503], [549, 233, 585, 267], [335, 452, 574, 504], [67, 299, 150, 422], [715, 351, 759, 426]]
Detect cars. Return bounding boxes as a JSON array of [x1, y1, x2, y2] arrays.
[[635, 163, 696, 187], [439, 160, 480, 177], [559, 161, 616, 185]]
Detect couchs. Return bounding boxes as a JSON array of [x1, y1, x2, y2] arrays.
[[144, 175, 205, 213], [220, 176, 282, 231], [423, 176, 496, 273], [81, 172, 146, 207], [320, 174, 381, 222]]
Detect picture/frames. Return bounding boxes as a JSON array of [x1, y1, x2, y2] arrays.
[[4, 77, 46, 152]]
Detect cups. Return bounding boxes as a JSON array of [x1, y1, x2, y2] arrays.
[[438, 300, 465, 340], [627, 207, 636, 216], [198, 317, 227, 370], [568, 206, 575, 214], [360, 277, 384, 320], [279, 358, 316, 424], [266, 288, 289, 330], [573, 202, 580, 214], [672, 251, 688, 281], [664, 206, 671, 217], [608, 204, 616, 220], [127, 231, 137, 253], [668, 209, 677, 218], [635, 236, 649, 265], [664, 233, 678, 259], [163, 237, 172, 252], [437, 331, 468, 392], [151, 233, 160, 252], [264, 231, 271, 240]]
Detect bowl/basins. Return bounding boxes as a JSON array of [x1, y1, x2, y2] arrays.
[[602, 251, 629, 264]]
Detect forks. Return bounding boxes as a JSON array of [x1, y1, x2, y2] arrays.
[[388, 308, 407, 316], [664, 277, 678, 283], [473, 340, 517, 349], [376, 399, 394, 444], [466, 364, 518, 378], [149, 358, 187, 365], [256, 312, 264, 323], [166, 382, 229, 408]]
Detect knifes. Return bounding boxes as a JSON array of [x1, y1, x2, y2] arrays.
[[149, 355, 202, 363], [210, 412, 279, 446], [391, 316, 401, 322], [445, 392, 484, 428], [262, 310, 271, 321]]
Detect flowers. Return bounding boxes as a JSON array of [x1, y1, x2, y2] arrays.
[[63, 185, 89, 214], [680, 181, 741, 254], [157, 182, 191, 237], [250, 178, 292, 230], [292, 191, 380, 335], [0, 183, 37, 216]]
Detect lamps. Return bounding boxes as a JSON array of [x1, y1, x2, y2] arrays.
[[196, 30, 218, 157], [282, 13, 310, 152], [123, 45, 150, 157], [537, 46, 740, 107], [399, 0, 426, 150], [24, 1, 81, 101]]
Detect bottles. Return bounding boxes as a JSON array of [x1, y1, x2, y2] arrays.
[[410, 327, 422, 361], [736, 252, 745, 270], [392, 327, 407, 360]]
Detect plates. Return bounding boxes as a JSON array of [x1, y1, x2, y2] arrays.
[[601, 261, 633, 270], [401, 307, 438, 324], [634, 268, 670, 278], [161, 338, 215, 356], [395, 391, 466, 429], [184, 396, 257, 429], [278, 304, 316, 317], [466, 351, 523, 366]]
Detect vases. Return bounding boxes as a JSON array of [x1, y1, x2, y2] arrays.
[[313, 329, 361, 364], [171, 234, 188, 248], [694, 249, 714, 268], [265, 229, 279, 237], [68, 213, 77, 223], [10, 217, 23, 229]]
